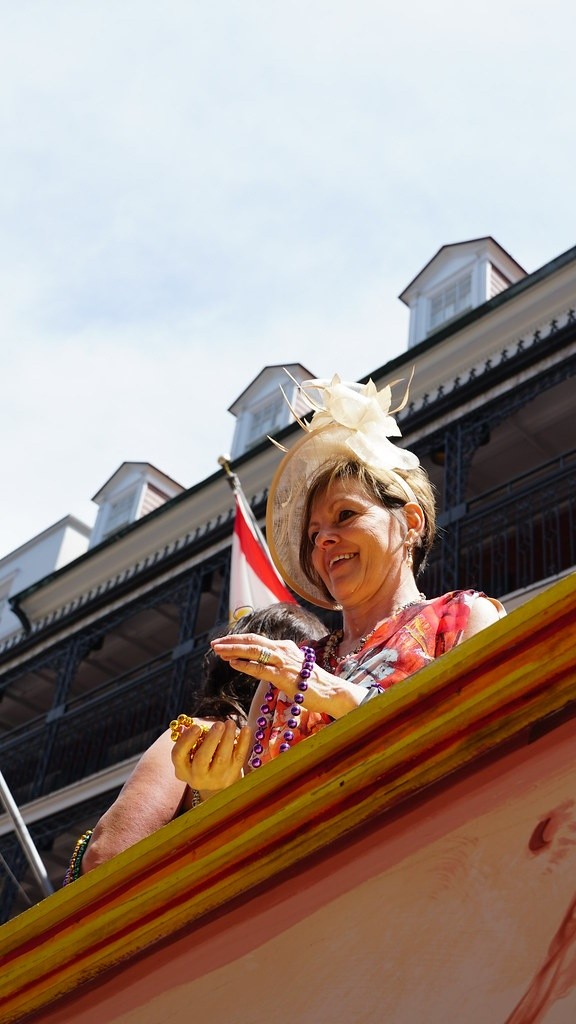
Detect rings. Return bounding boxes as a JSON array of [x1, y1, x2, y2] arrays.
[[260, 647, 270, 663]]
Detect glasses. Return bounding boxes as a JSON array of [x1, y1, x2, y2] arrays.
[[233, 605, 254, 622]]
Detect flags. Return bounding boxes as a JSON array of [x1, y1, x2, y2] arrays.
[[230, 478, 296, 620]]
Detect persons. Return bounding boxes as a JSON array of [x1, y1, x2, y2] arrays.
[[172, 384, 508, 809], [64, 604, 323, 882]]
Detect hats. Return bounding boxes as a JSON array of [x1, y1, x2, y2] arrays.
[[265, 365, 419, 609]]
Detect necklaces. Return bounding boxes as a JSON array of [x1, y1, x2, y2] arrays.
[[252, 647, 316, 767], [63, 829, 93, 887], [170, 713, 236, 765], [322, 591, 425, 672]]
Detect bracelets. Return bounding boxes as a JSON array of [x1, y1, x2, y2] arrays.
[[189, 790, 199, 807]]
